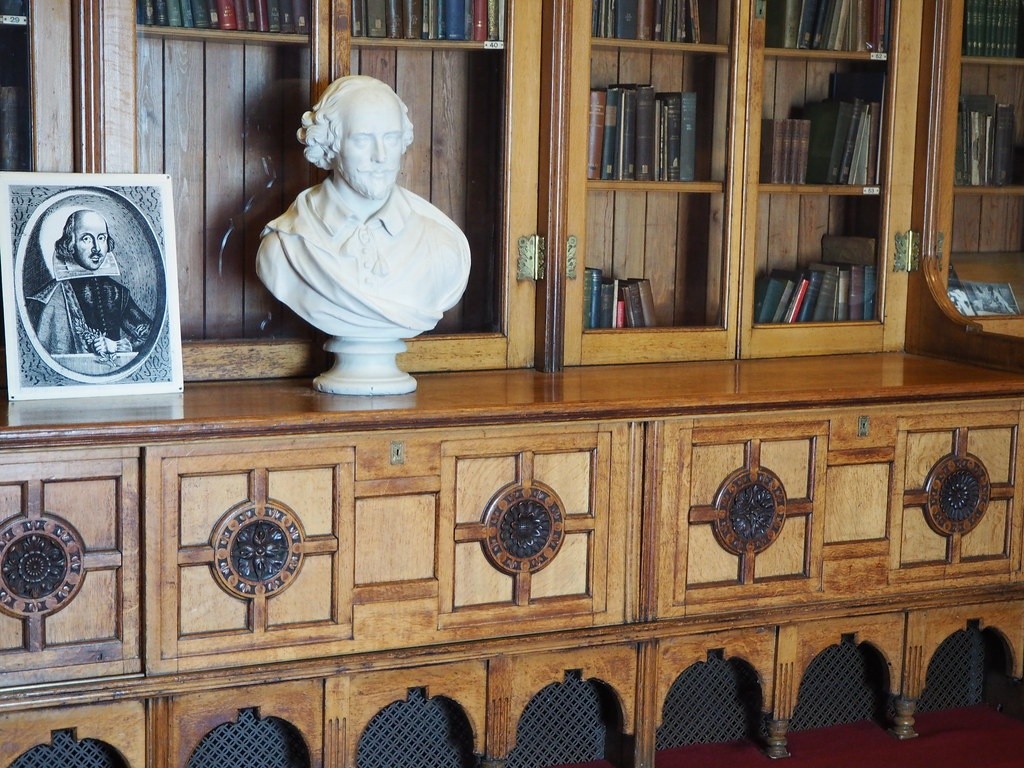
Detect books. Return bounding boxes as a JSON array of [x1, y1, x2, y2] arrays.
[[592, 0, 701, 44], [956, 93, 1014, 186], [137, 0, 312, 34], [583, 268, 656, 329], [753, 232, 876, 324], [587, 83, 696, 183], [351, 0, 505, 41], [765, 0, 890, 52], [965, 0, 1024, 56], [761, 68, 885, 187]]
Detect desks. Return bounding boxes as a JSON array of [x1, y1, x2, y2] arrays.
[[0, 351, 1023, 768]]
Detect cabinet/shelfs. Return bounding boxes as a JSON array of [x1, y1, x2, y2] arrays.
[[1, 0, 1023, 381]]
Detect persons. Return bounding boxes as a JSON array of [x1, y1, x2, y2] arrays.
[[946, 283, 1016, 317], [253, 77, 472, 341]]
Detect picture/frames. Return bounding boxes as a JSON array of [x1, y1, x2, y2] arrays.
[[1, 171, 185, 402]]
